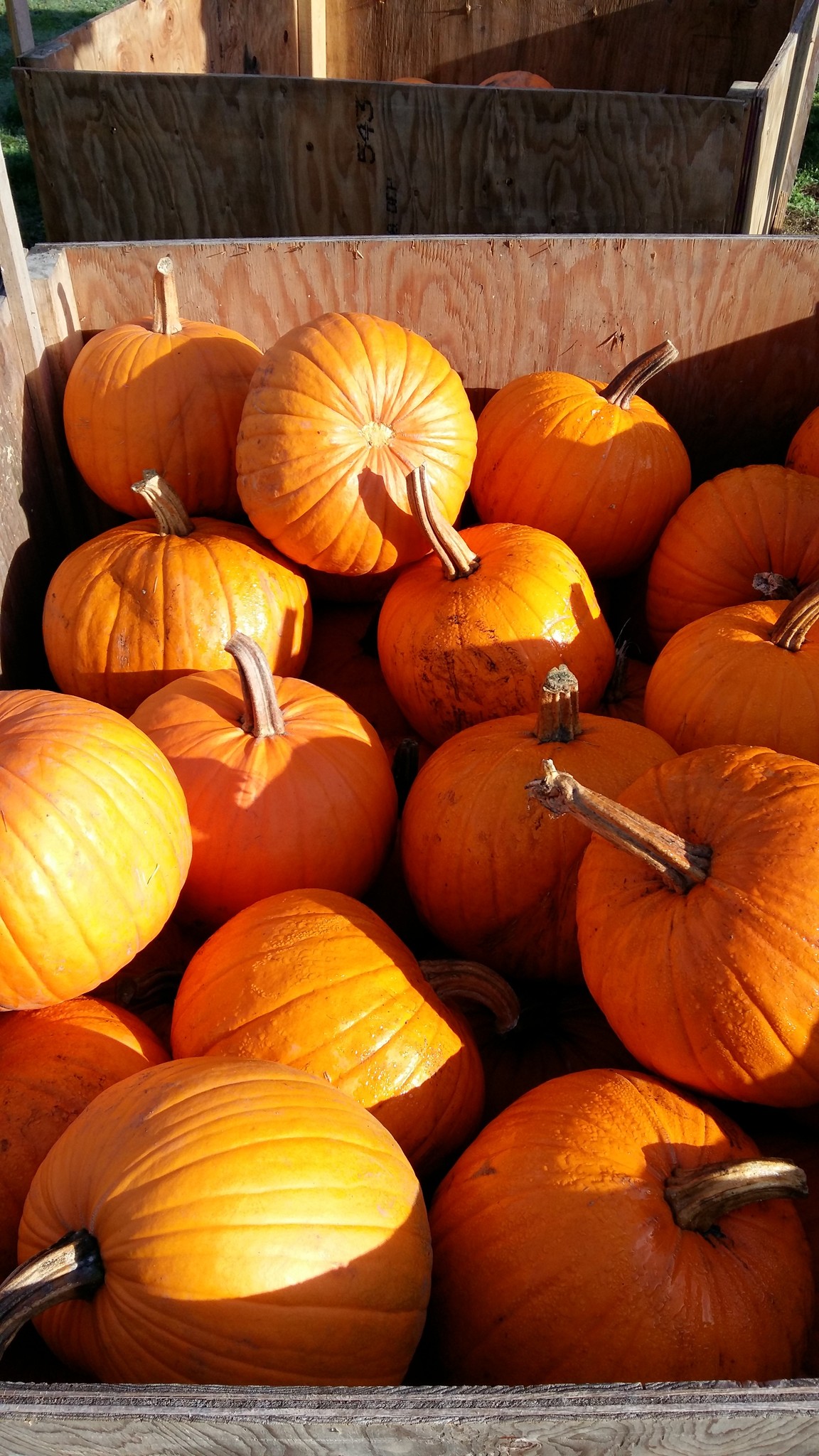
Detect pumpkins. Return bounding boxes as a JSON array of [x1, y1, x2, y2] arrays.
[[235, 310, 479, 578], [424, 1069, 814, 1384], [645, 578, 819, 762], [467, 338, 692, 577], [644, 461, 819, 643], [42, 469, 312, 722], [127, 633, 397, 929], [0, 1057, 434, 1385], [169, 888, 519, 1171], [62, 256, 265, 521], [782, 405, 819, 475], [525, 744, 819, 1110], [378, 463, 618, 748], [1, 685, 191, 1013], [1, 997, 171, 1256], [399, 664, 682, 985]]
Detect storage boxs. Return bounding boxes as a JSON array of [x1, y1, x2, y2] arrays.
[[10, 4, 819, 238], [2, 236, 819, 1456]]
